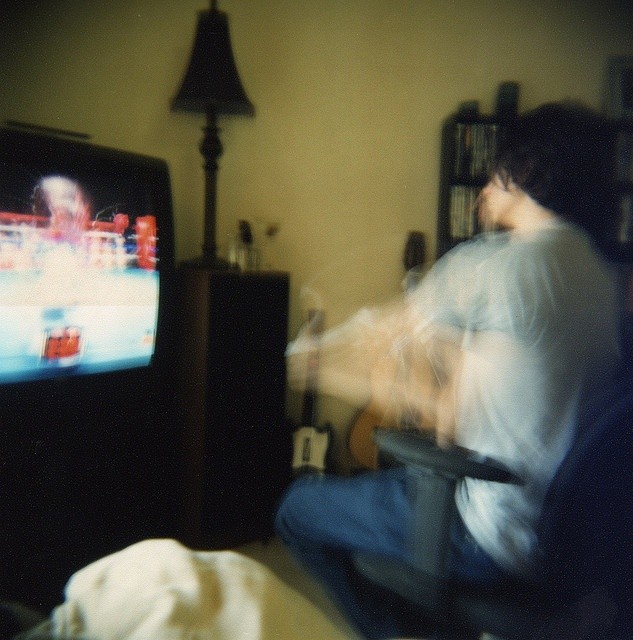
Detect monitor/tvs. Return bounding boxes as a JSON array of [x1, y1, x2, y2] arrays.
[[2, 130, 176, 509]]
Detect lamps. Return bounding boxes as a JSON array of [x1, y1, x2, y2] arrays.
[[169, 0, 255, 269]]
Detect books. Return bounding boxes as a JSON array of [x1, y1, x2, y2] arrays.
[[450, 183, 499, 238], [452, 121, 498, 178]]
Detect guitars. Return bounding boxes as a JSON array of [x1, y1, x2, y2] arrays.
[[344, 231, 442, 471], [288, 309, 332, 474]]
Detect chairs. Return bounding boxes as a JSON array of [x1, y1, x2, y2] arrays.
[[336, 388, 632, 637]]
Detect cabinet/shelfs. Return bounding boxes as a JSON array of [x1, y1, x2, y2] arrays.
[[438, 114, 501, 244]]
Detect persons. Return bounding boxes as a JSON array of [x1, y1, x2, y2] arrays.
[[272, 100, 631, 640]]
